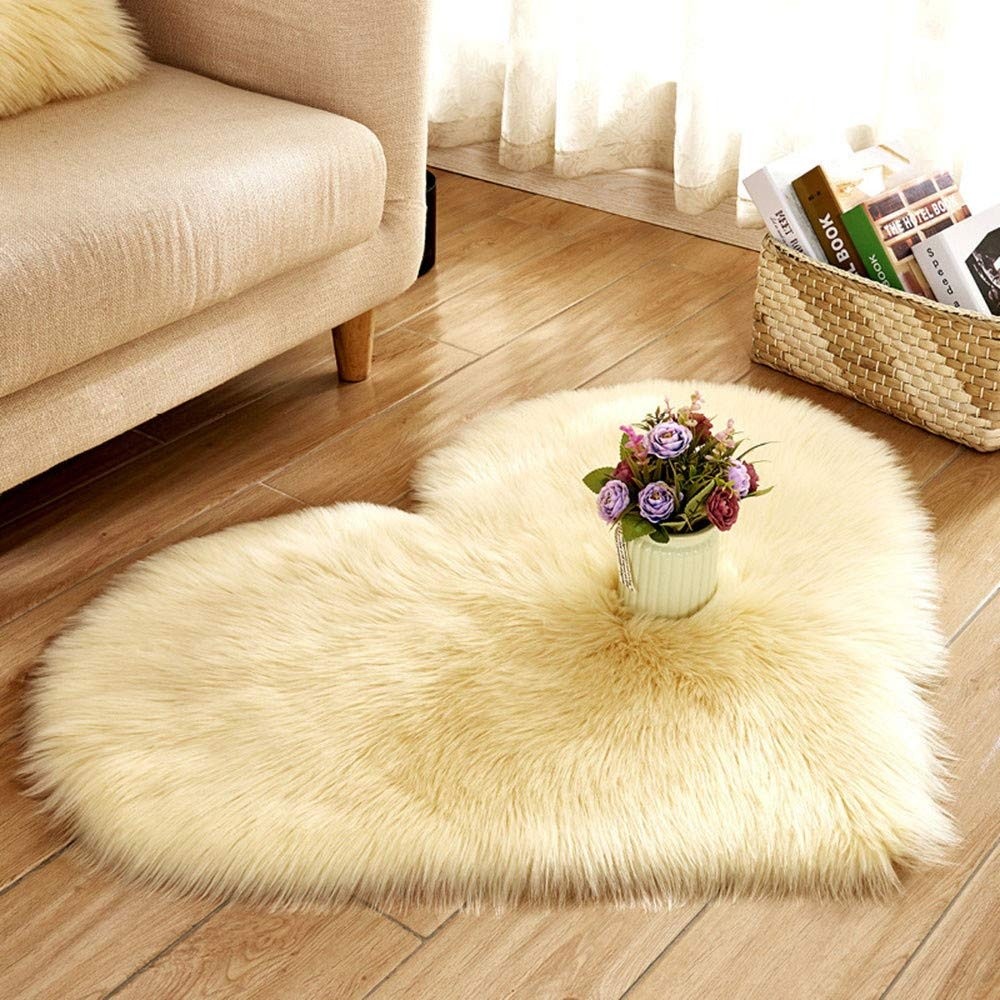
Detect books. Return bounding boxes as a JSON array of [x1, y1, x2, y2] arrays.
[[743, 132, 854, 266], [792, 144, 916, 279], [842, 166, 971, 302], [912, 201, 1000, 316]]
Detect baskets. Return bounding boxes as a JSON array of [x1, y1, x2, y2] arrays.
[[750, 231, 999, 453]]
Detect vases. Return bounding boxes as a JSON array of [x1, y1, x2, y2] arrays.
[[619, 523, 718, 619]]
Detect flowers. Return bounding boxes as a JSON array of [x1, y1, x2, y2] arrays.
[[583, 391, 781, 593]]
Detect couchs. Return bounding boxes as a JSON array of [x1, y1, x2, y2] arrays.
[[0, 0, 430, 493]]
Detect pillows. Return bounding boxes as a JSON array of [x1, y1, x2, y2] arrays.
[[0, 0, 148, 118]]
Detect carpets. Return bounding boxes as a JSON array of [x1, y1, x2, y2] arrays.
[[26, 385, 962, 906]]
